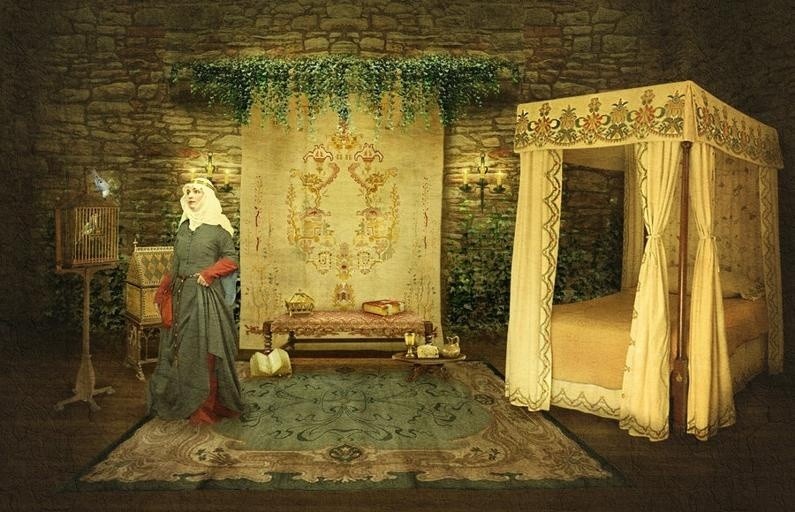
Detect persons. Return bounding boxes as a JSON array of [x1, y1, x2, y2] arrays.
[[151, 172, 247, 427]]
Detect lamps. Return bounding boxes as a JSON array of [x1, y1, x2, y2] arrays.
[[189, 151, 233, 193], [460, 150, 506, 213]]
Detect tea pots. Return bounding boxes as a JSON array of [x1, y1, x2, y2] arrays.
[[436, 335, 462, 359]]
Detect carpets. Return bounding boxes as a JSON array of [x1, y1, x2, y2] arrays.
[[63, 356, 635, 492]]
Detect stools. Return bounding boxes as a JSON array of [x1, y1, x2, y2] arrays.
[[121, 246, 174, 382]]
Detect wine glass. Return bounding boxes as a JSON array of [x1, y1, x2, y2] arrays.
[[403, 332, 417, 360]]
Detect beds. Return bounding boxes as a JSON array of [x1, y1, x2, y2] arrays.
[[504, 80, 785, 442]]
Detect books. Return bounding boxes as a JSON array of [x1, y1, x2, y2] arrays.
[[362, 296, 405, 318], [249, 346, 293, 380]]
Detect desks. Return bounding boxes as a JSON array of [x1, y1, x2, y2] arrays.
[[262, 308, 467, 379]]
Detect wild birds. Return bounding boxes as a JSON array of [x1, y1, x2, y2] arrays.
[[77, 213, 100, 244]]
